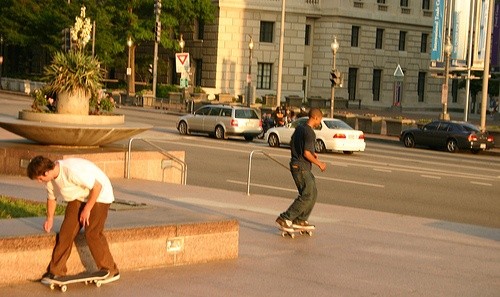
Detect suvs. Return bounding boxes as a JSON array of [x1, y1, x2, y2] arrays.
[[176, 103, 265, 141]]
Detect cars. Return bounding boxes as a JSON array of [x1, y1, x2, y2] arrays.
[[399, 120, 495, 154], [266, 117, 366, 154]]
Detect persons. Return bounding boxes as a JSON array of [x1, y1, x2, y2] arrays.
[[261, 106, 298, 133], [25, 155, 121, 283], [275, 107, 326, 232], [296, 106, 308, 117]]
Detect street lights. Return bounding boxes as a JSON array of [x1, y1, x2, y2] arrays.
[[178, 34, 185, 105], [246, 37, 252, 108], [124, 35, 134, 106], [329, 36, 340, 118]]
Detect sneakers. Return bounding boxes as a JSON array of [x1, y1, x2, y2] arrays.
[[292, 219, 314, 229], [276, 217, 294, 232]]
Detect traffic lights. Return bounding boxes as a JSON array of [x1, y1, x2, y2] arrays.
[[329, 70, 341, 86]]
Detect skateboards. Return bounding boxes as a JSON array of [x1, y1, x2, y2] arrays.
[[278, 224, 317, 239], [40, 270, 110, 293]]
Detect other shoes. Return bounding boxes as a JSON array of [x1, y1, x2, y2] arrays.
[[94, 273, 120, 284], [41, 273, 58, 284]]
